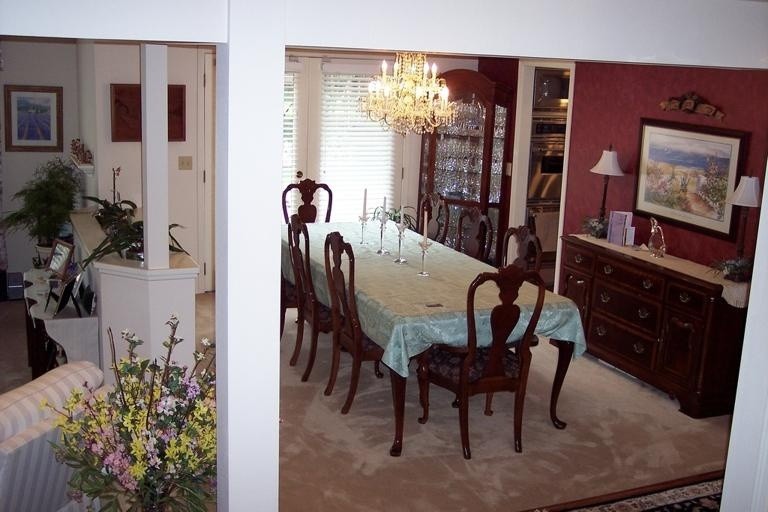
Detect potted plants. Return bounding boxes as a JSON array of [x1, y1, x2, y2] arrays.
[[82, 214, 191, 269], [2, 156, 84, 267], [83, 167, 139, 236]]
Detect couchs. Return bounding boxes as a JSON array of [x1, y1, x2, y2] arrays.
[[0, 360, 115, 512]]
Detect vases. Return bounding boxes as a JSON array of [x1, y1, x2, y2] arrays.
[[141, 505, 167, 512]]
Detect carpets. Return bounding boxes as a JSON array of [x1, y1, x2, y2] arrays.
[[520, 470, 726, 512]]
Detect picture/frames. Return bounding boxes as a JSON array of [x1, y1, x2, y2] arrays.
[[71, 268, 86, 299], [51, 278, 83, 319], [110, 82, 187, 143], [44, 237, 76, 281], [632, 117, 753, 248], [5, 84, 63, 152]]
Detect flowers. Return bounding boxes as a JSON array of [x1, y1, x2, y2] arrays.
[[39, 312, 217, 512]]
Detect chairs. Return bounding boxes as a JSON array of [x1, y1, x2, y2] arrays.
[[282, 178, 333, 225], [416, 190, 450, 245], [287, 214, 346, 382], [279, 273, 299, 340], [500, 225, 542, 282], [324, 231, 386, 415], [415, 266, 546, 461], [452, 203, 493, 263]]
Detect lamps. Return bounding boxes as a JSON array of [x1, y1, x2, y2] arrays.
[[357, 49, 458, 139], [586, 142, 626, 239], [725, 172, 763, 260]]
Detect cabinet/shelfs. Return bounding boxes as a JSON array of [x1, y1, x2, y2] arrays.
[[557, 233, 751, 420], [24, 208, 201, 387]]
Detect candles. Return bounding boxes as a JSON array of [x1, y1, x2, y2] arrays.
[[362, 188, 367, 218], [424, 210, 427, 246], [382, 197, 388, 219], [400, 206, 405, 225]]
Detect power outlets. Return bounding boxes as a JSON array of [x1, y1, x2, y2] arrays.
[[177, 155, 193, 170]]
